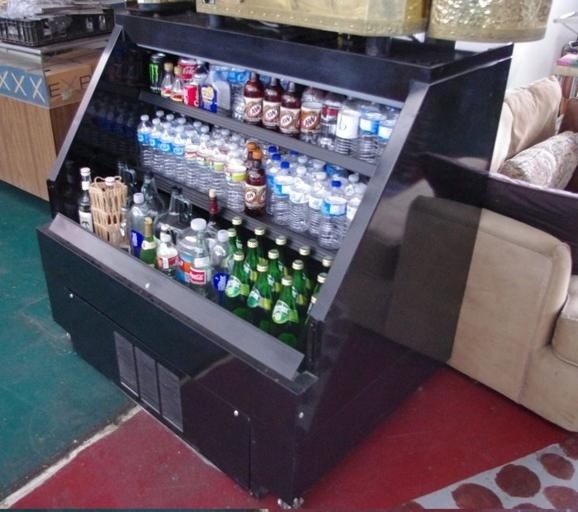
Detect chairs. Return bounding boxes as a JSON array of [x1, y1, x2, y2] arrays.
[[445, 76, 578, 436]]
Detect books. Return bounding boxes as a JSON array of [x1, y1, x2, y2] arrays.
[[551, 52, 578, 99]]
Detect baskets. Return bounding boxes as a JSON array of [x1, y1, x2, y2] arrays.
[[1, 10, 113, 47]]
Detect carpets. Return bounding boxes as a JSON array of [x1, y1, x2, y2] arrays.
[[400, 442, 577, 509]]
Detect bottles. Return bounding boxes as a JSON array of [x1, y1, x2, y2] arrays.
[[77, 54, 402, 371]]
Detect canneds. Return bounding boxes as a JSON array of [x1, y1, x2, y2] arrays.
[[148, 53, 166, 94], [182, 83, 198, 108]]
